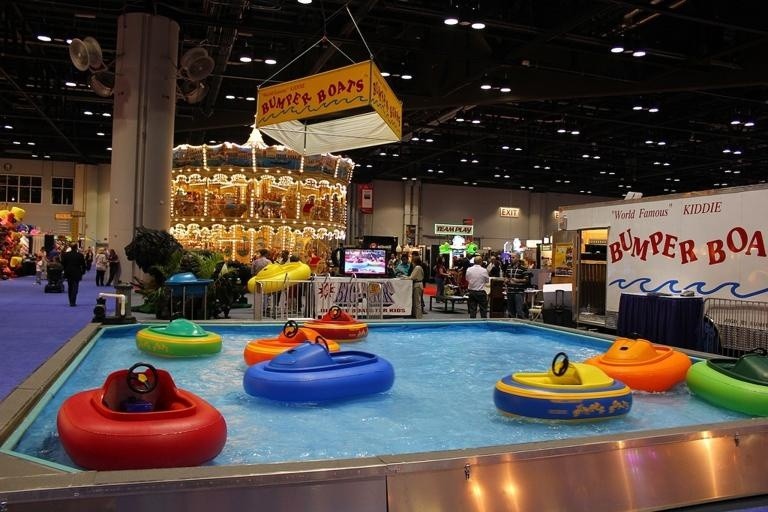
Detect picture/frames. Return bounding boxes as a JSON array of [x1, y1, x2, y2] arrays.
[[554, 243, 574, 277]]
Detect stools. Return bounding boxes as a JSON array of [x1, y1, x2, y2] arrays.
[[525, 289, 540, 319]]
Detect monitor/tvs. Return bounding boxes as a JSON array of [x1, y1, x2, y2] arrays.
[[339, 247, 388, 277]]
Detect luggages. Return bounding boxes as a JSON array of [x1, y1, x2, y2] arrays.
[[542, 290, 572, 328]]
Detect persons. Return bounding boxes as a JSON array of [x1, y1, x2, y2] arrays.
[[251, 249, 307, 318], [84, 247, 93, 271], [105, 247, 120, 286], [34, 240, 60, 283], [388, 250, 429, 320], [61, 243, 86, 306], [95, 247, 108, 287], [434, 249, 529, 319]]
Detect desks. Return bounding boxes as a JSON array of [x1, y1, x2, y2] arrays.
[[616, 293, 704, 347], [429, 294, 470, 314]]
